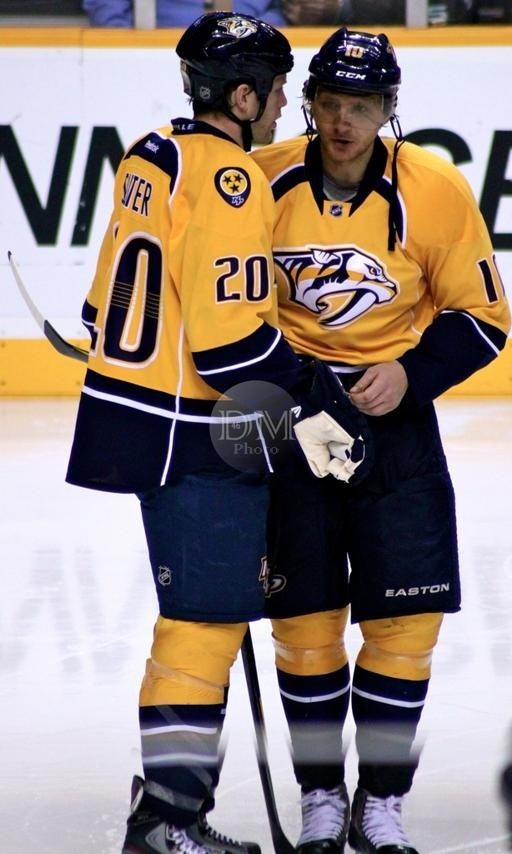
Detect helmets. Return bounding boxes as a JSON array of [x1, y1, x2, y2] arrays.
[[301, 27, 401, 133], [175, 11, 293, 126]]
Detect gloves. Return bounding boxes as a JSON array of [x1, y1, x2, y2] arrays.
[[290, 352, 371, 483]]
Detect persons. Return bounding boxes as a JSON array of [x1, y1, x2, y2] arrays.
[[65, 11, 368, 854], [244, 28, 512, 854]]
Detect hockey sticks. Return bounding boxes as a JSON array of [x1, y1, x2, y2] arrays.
[[8, 252, 364, 463], [241, 623, 299, 854]]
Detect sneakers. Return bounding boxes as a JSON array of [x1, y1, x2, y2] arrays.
[[348, 787, 419, 853], [122, 810, 262, 854], [294, 780, 352, 854]]
